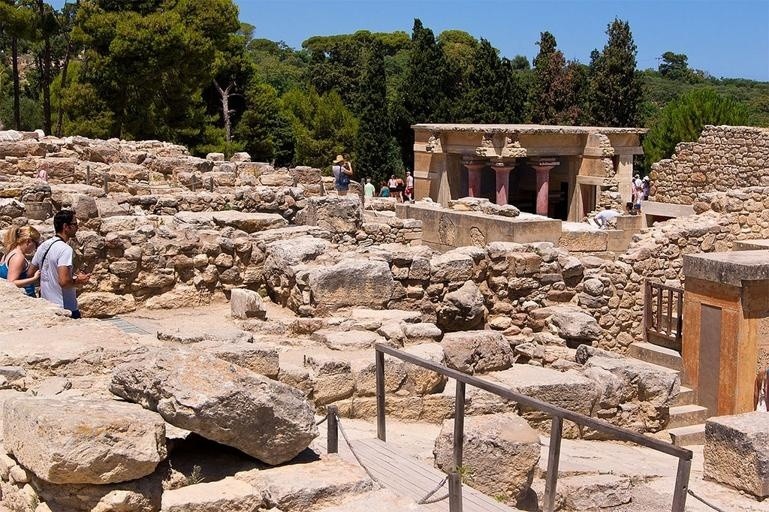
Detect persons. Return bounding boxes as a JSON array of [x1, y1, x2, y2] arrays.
[[33, 163, 48, 182], [0, 223, 41, 300], [363, 178, 376, 198], [630, 174, 650, 214], [330, 155, 354, 196], [388, 175, 397, 197], [402, 170, 415, 200], [378, 182, 389, 198], [25, 209, 93, 308], [396, 175, 406, 202], [593, 202, 620, 227]]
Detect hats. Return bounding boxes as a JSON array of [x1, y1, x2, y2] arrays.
[[332, 155, 347, 163]]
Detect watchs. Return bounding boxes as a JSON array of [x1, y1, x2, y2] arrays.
[[71, 274, 78, 284]]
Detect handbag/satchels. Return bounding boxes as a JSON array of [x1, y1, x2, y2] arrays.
[[338, 166, 351, 187]]
